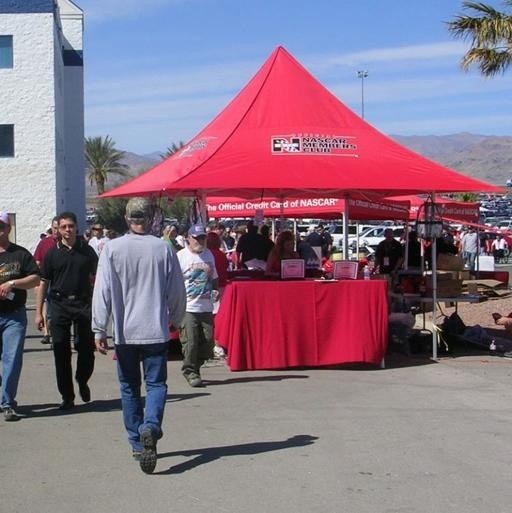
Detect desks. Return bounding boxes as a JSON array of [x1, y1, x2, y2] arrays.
[[385, 288, 489, 359], [221, 276, 390, 371]]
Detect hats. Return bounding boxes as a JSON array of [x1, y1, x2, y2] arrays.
[[126, 197, 154, 223], [188, 224, 208, 238], [0, 212, 9, 225]]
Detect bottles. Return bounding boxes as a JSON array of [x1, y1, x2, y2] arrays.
[[419, 276, 427, 296], [227, 261, 233, 271], [489, 338, 497, 356], [320, 272, 333, 279], [363, 265, 371, 281], [213, 339, 225, 366]]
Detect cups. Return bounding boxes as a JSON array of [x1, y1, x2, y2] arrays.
[[467, 281, 478, 297]]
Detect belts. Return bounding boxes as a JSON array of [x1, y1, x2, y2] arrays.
[[56, 293, 87, 300]]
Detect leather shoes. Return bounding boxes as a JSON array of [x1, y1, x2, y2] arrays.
[[76, 378, 90, 401], [59, 401, 74, 409]]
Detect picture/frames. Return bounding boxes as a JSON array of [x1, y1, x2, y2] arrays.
[[279, 257, 305, 278], [333, 260, 358, 279]]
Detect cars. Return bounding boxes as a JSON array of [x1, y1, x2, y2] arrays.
[[483, 214, 511, 230], [295, 221, 369, 244], [348, 225, 404, 249]]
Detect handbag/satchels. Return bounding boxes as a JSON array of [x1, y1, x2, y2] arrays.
[[416, 202, 443, 238]]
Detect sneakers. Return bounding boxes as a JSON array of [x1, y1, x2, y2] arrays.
[[4, 407, 18, 421], [183, 370, 202, 386], [133, 425, 157, 472]]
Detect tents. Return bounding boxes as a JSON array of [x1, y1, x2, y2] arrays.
[[205, 192, 480, 270]]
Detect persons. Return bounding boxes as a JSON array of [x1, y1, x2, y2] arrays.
[[205, 221, 333, 272], [34, 216, 61, 349], [374, 227, 490, 287], [30, 208, 102, 410], [491, 234, 508, 265], [85, 223, 116, 256], [160, 223, 229, 386], [90, 194, 188, 474], [1, 211, 44, 423]]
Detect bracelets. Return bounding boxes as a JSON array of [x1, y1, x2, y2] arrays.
[[8, 279, 16, 288]]
